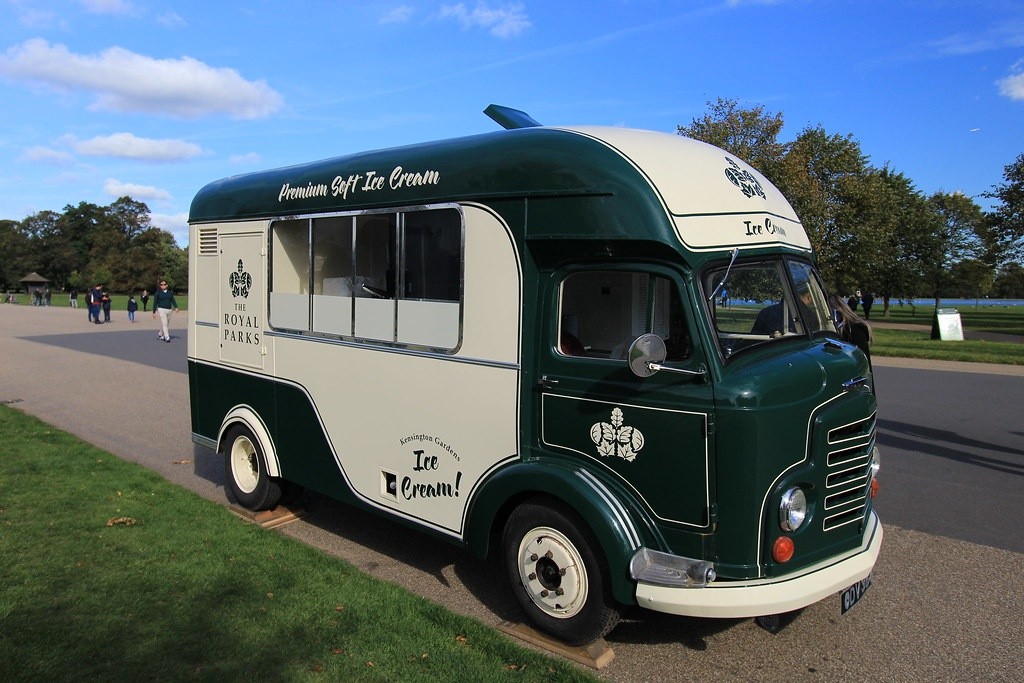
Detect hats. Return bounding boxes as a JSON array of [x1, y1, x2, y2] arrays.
[[787, 261, 810, 292]]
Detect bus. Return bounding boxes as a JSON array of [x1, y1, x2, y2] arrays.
[[185, 104, 884, 648]]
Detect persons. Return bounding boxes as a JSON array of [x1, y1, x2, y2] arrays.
[[847, 295, 857, 312], [720, 289, 729, 308], [751, 281, 820, 334], [69, 290, 79, 309], [140, 289, 150, 312], [153, 280, 179, 343], [32, 288, 51, 306], [861, 290, 874, 320], [85, 283, 112, 324], [128, 295, 138, 323]]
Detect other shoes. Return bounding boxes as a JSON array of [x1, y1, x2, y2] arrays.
[[165, 337, 171, 343], [158, 335, 165, 341]]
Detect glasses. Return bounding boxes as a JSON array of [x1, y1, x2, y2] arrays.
[[159, 284, 167, 286]]
